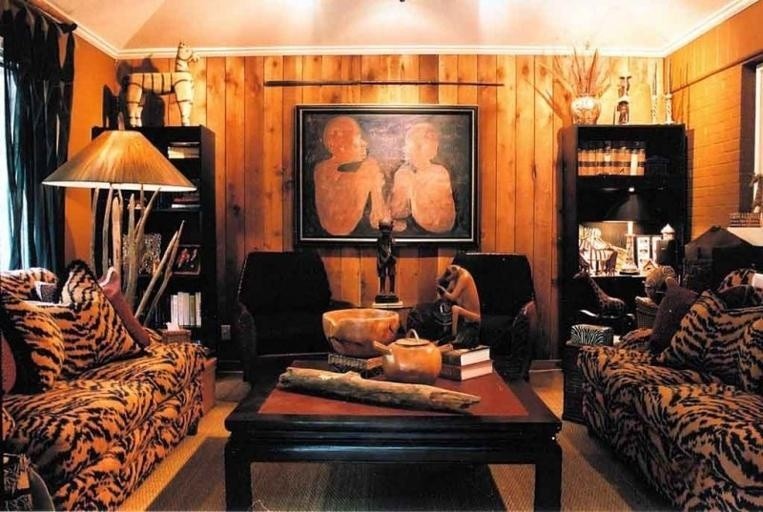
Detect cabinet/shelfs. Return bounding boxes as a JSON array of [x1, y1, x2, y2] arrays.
[[555, 122, 690, 360], [90, 124, 219, 382]]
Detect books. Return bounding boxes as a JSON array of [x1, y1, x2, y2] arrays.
[[439, 358, 496, 383], [432, 339, 491, 367], [127, 139, 202, 211], [132, 285, 202, 329]]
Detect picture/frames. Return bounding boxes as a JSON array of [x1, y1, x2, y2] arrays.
[[293, 103, 481, 249]]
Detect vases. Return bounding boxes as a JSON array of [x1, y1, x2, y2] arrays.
[[569, 93, 602, 126]]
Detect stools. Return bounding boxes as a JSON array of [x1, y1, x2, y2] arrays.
[[198, 356, 219, 417]]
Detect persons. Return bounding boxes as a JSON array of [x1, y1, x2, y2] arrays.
[[374, 215, 397, 294]]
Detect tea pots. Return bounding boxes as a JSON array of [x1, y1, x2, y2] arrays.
[[369, 328, 453, 383]]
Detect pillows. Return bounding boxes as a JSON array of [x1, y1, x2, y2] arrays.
[[1, 258, 152, 393], [649, 276, 763, 390]]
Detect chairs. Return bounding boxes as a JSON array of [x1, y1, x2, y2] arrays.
[[235, 249, 357, 388], [406, 252, 541, 382]]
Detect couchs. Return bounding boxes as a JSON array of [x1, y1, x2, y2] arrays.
[[0, 266, 206, 512], [576, 268, 763, 512]]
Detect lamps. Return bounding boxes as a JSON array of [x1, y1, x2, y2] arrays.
[[41, 129, 199, 277]]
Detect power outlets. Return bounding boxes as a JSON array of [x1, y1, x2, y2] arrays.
[[221, 324, 231, 340]]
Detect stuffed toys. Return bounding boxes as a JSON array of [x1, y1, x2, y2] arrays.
[[747, 170, 762, 214]]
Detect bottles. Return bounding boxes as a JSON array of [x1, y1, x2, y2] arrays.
[[578, 141, 646, 176]]
